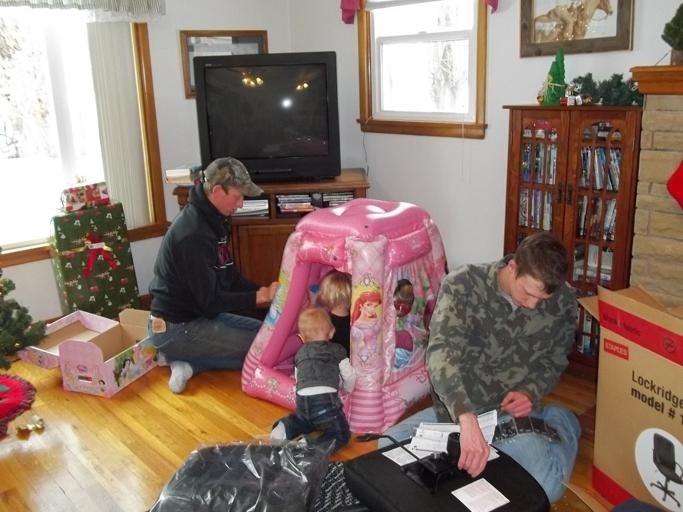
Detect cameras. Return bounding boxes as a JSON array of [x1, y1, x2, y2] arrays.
[[417, 431, 487, 490]]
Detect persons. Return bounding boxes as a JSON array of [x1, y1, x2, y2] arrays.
[[145, 154, 281, 393], [349, 273, 385, 392], [267, 307, 356, 464], [378, 230, 582, 507], [309, 268, 351, 362]]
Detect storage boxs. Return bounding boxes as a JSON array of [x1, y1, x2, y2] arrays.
[[576, 286, 683, 512], [16, 306, 153, 398]]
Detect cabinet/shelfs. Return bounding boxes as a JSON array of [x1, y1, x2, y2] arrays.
[[500, 106, 643, 365], [173, 167, 370, 320]]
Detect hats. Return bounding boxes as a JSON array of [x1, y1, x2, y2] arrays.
[[204, 157, 263, 201]]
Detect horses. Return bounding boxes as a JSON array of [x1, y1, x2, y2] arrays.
[[534, 0, 614, 43]]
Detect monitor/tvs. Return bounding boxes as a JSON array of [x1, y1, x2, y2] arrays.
[[193, 51, 342, 184]]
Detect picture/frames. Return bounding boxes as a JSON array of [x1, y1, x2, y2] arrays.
[[519, 0, 634, 60], [180, 29, 269, 98]]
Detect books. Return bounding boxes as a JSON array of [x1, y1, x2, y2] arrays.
[[274, 191, 354, 215], [516, 122, 623, 358]]
